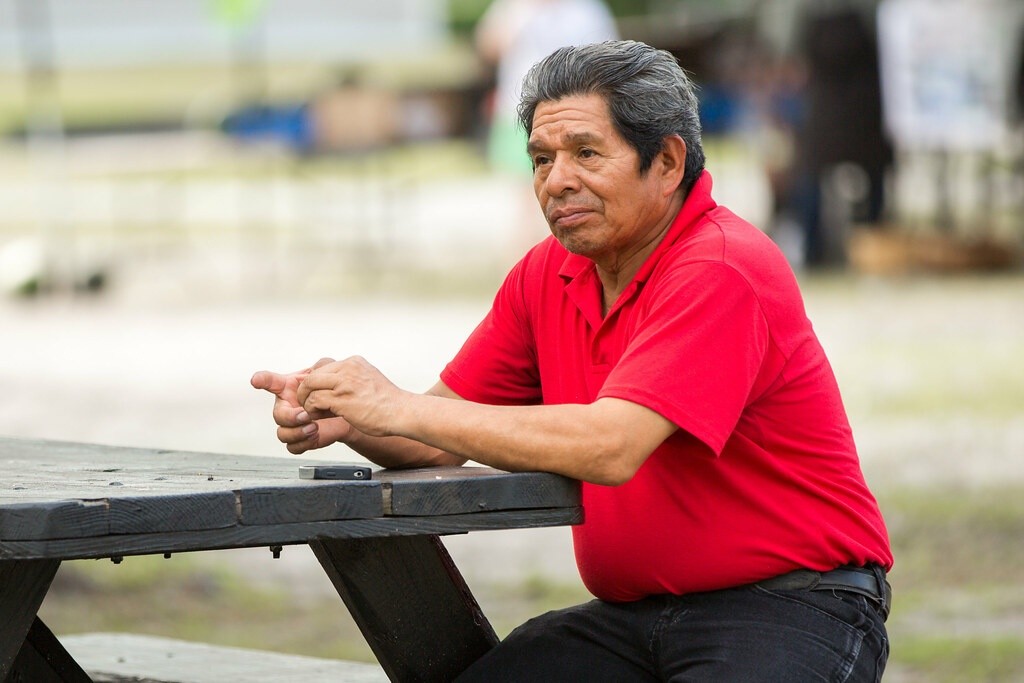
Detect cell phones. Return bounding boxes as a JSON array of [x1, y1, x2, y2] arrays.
[[298, 465, 372, 481]]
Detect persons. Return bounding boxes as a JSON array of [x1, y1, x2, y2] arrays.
[[251, 39, 894, 683], [472, 0, 616, 177]]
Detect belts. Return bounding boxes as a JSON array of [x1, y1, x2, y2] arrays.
[[757, 568, 892, 623]]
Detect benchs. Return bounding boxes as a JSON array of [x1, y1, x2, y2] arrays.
[[55, 631, 393, 683]]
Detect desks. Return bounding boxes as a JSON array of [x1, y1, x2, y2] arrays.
[[0, 439, 584, 683]]
